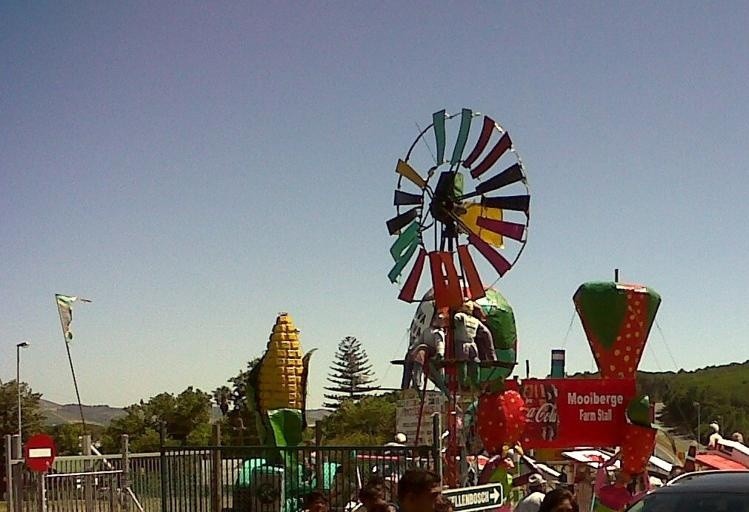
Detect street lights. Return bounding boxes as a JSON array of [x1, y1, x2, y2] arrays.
[[15, 340, 30, 459], [693, 401, 702, 452]]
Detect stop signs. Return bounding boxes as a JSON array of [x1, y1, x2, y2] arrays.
[[24, 433, 56, 473]]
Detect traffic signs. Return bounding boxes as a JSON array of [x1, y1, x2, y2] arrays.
[[440, 482, 504, 511]]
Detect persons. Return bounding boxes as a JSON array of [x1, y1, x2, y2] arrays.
[[708, 421, 722, 449], [731, 432, 744, 444], [452, 298, 498, 392], [400, 306, 449, 391], [295, 431, 579, 512]]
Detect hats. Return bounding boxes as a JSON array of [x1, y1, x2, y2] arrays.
[[528, 473, 547, 486]]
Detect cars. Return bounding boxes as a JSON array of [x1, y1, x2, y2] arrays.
[[624, 468, 749, 510]]
